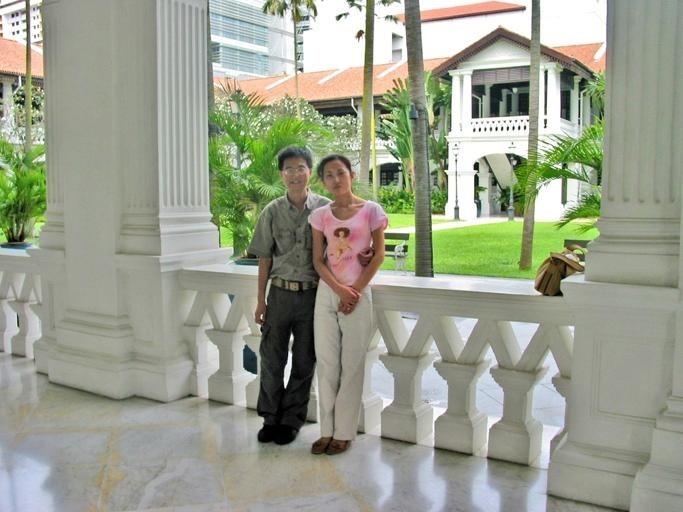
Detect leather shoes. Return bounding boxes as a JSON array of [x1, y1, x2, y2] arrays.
[[327, 439, 348, 455], [312, 437, 332, 454]]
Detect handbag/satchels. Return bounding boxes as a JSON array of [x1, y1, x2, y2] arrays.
[[535, 244, 585, 296]]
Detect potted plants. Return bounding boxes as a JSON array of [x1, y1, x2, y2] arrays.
[[0, 137, 46, 249]]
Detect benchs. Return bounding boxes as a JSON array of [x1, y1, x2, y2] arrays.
[[383, 232, 409, 271]]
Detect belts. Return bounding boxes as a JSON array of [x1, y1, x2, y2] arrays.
[[272, 278, 317, 291]]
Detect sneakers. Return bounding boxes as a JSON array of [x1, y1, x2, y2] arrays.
[[258, 424, 277, 443], [273, 425, 297, 445]]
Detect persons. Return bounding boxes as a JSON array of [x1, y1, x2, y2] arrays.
[[248, 144, 375, 446], [307, 155, 388, 457]]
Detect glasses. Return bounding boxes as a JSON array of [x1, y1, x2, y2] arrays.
[[285, 167, 307, 175]]
